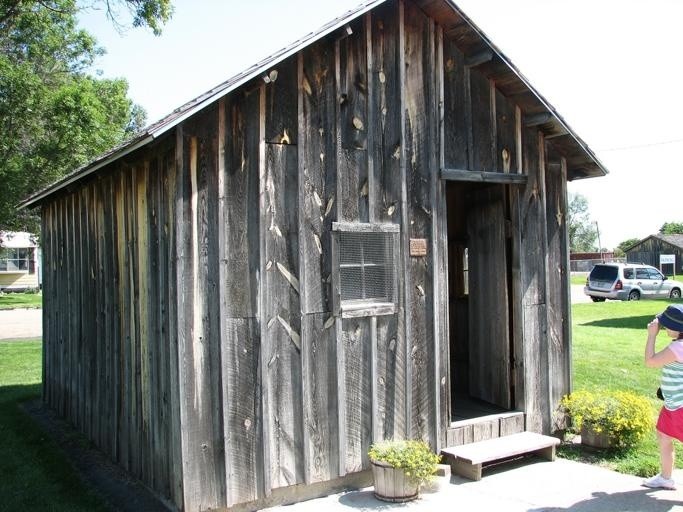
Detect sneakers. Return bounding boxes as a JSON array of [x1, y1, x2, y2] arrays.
[[642, 473, 675, 490]]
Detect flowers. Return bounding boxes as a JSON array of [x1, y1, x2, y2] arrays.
[[560, 386, 657, 452], [368, 437, 444, 505]]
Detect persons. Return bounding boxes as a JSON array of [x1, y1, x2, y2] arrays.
[[642, 305, 683, 490]]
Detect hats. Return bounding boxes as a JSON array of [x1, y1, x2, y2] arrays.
[[655, 304, 683, 331]]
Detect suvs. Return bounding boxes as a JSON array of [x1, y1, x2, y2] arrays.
[[583, 260, 683, 301]]
